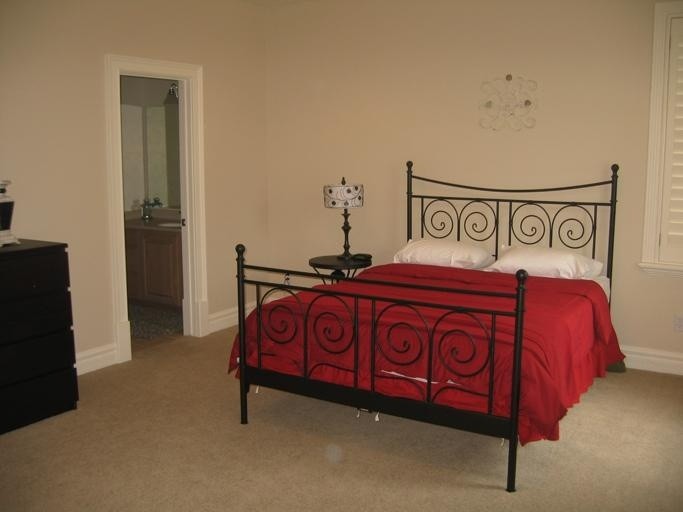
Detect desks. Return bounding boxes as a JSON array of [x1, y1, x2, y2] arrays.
[[309, 256, 371, 285]]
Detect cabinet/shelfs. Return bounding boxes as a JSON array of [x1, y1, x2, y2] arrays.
[[126, 229, 184, 312], [0, 238, 78, 434]]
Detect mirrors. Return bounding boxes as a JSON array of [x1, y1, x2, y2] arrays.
[[147, 104, 180, 208]]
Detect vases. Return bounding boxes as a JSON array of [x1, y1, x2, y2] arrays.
[[0, 180, 21, 248]]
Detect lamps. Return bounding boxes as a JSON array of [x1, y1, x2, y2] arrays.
[[168, 83, 179, 101], [324, 177, 364, 260]]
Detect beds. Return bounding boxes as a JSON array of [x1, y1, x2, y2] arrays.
[[235, 162, 619, 492]]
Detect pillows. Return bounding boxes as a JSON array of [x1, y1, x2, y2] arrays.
[[393, 239, 604, 280]]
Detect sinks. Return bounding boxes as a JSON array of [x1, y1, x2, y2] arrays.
[[159, 222, 182, 228]]
